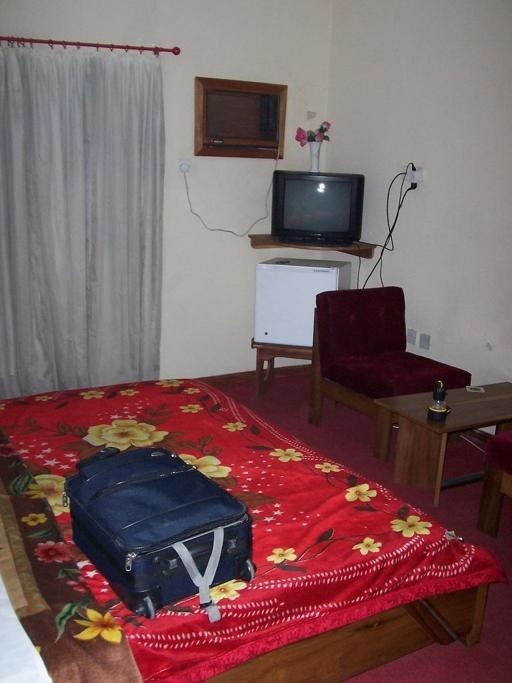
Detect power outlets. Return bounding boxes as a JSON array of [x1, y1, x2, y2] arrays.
[[401, 165, 424, 189]]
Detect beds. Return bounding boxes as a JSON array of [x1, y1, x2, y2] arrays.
[[0, 378, 494, 682]]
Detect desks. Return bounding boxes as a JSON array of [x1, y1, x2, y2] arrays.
[[373, 378, 512, 507], [251, 336, 338, 410]]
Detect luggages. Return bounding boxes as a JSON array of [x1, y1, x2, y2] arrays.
[[60, 445, 257, 624]]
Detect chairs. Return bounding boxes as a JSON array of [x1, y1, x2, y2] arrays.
[[308, 286, 471, 465]]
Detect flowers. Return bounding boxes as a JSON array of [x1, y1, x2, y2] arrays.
[[295, 121, 330, 147]]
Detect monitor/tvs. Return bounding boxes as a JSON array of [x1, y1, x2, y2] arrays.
[[271, 169, 365, 247]]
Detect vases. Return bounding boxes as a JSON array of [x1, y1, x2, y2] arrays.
[[308, 141, 321, 172]]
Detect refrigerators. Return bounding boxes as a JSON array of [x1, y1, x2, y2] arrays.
[[253, 258, 352, 350]]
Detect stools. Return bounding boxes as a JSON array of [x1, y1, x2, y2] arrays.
[[476, 430, 512, 538]]
